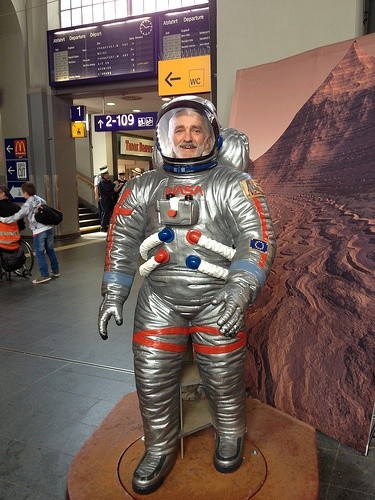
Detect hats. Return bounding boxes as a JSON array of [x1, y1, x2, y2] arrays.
[[99, 166, 108, 173], [132, 168, 141, 175]]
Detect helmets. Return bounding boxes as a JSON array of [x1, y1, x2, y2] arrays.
[[156, 96, 223, 167]]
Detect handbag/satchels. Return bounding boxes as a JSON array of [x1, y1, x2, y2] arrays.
[[34, 204, 63, 226]]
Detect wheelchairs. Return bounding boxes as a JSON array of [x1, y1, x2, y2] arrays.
[[0, 238, 35, 283]]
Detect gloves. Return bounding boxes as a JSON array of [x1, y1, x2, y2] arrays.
[[212, 284, 252, 339], [98, 293, 125, 341]]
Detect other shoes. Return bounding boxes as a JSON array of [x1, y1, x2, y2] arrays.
[[215, 434, 244, 472], [33, 276, 52, 283], [49, 271, 61, 276], [133, 450, 177, 495]]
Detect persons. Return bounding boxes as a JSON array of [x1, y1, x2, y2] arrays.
[[93, 164, 144, 232], [0, 181, 61, 284], [97, 96, 277, 494], [0, 183, 26, 233]]
